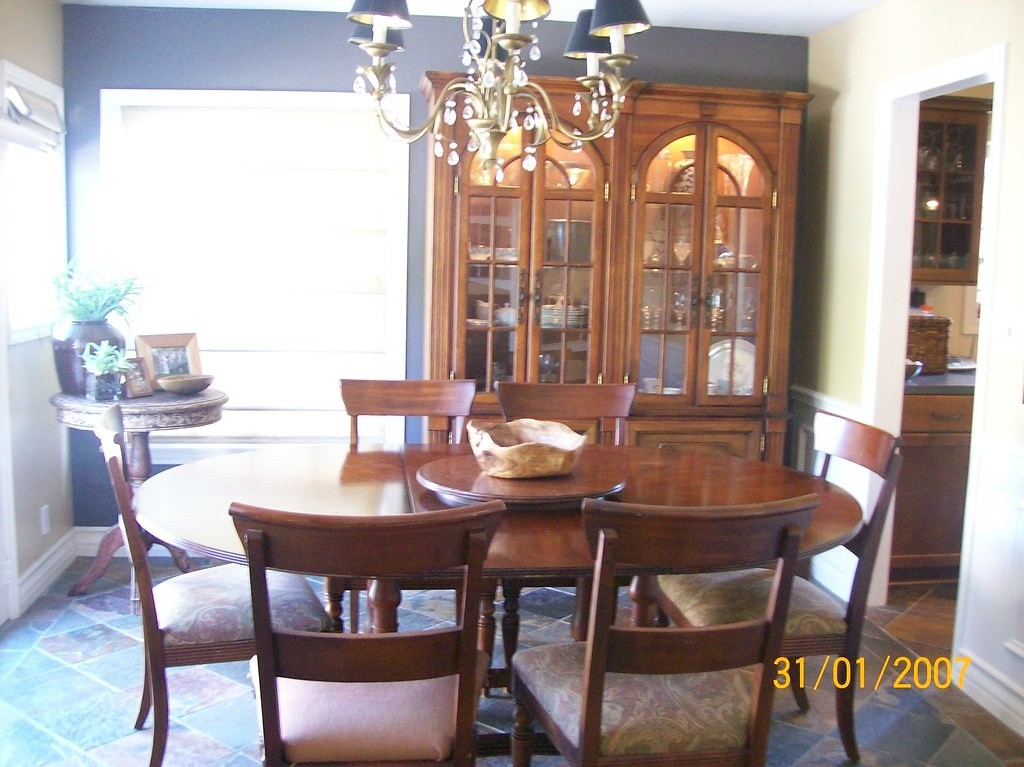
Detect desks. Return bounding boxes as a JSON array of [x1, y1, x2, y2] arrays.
[[128, 443, 863, 756], [48, 387, 228, 597]]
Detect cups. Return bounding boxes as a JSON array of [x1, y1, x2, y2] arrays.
[[706, 383, 716, 394], [641, 377, 658, 391], [740, 385, 752, 393], [718, 154, 756, 196], [643, 306, 661, 330], [709, 307, 726, 332]]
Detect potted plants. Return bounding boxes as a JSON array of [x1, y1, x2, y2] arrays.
[[48, 256, 143, 400], [78, 339, 136, 403]]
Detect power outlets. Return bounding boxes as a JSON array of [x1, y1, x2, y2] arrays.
[[41, 504, 51, 536]]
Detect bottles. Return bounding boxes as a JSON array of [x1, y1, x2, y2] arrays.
[[649, 149, 695, 193]]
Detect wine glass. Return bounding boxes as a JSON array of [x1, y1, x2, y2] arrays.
[[541, 352, 556, 383], [674, 242, 690, 267], [645, 229, 664, 266], [670, 286, 687, 330]]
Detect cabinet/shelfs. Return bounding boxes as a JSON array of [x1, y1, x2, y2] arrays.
[[887, 395, 974, 586], [418, 69, 816, 466], [910, 95, 992, 286]]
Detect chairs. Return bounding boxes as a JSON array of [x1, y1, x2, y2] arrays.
[[493, 381, 637, 695], [323, 379, 491, 698], [510, 492, 822, 767], [92, 404, 337, 767], [227, 500, 506, 767], [629, 410, 905, 761]]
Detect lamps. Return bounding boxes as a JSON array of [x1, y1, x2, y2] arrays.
[[345, 0, 651, 183]]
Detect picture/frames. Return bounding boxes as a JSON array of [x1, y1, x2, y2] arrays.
[[134, 333, 202, 392]]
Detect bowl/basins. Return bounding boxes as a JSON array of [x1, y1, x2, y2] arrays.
[[469, 246, 518, 261], [717, 259, 761, 270], [474, 303, 497, 320], [156, 374, 214, 396], [466, 416, 589, 480], [906, 363, 922, 383], [663, 387, 681, 394], [494, 303, 516, 325]]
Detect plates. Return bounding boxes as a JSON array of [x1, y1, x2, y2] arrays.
[[466, 319, 499, 326], [541, 305, 589, 329], [639, 389, 657, 394], [708, 339, 755, 394]]
